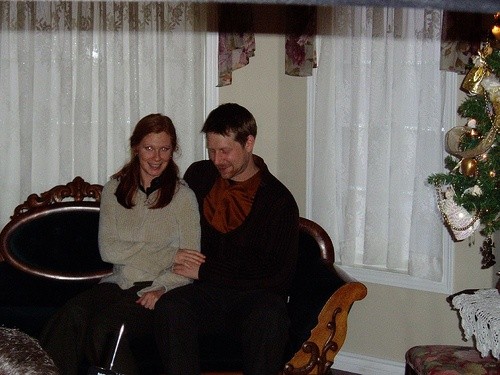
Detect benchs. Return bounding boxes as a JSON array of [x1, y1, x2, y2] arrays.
[[0, 176, 368, 375]]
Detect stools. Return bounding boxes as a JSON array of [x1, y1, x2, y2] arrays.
[[404, 346, 500, 375]]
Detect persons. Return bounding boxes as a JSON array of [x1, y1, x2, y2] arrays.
[[154, 102, 299, 375], [40, 113, 205, 375]]
